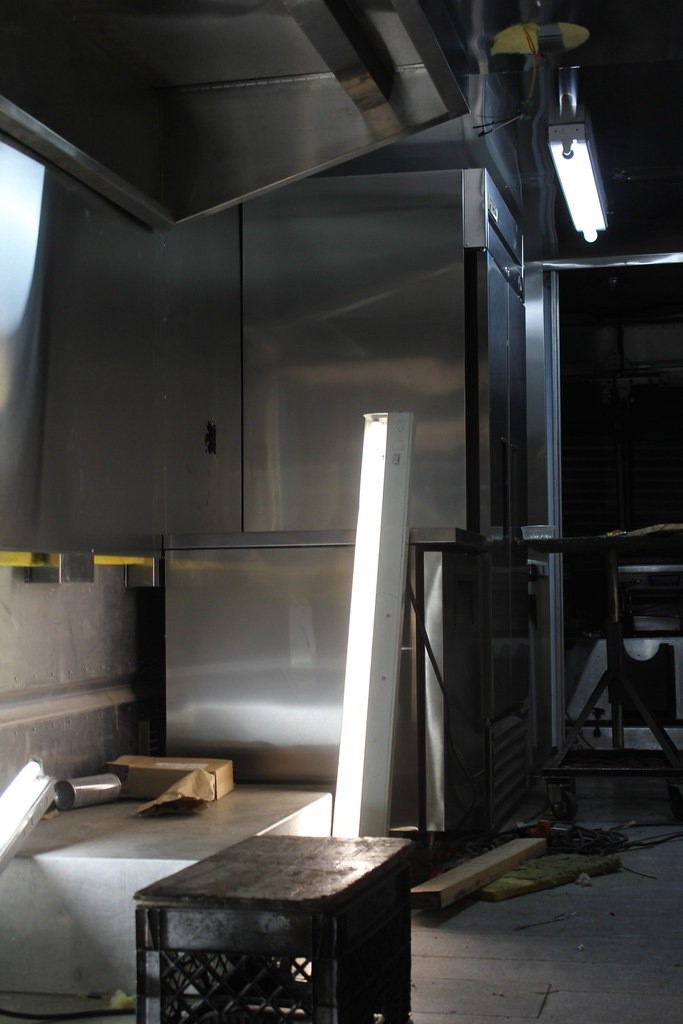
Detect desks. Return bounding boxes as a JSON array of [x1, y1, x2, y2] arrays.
[[133, 836, 416, 1024]]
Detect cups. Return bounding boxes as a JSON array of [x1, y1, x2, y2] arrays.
[[53, 773, 122, 810]]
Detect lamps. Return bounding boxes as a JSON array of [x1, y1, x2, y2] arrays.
[[548, 123, 609, 243]]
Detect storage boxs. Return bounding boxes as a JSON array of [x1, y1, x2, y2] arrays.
[[105, 755, 234, 801]]
